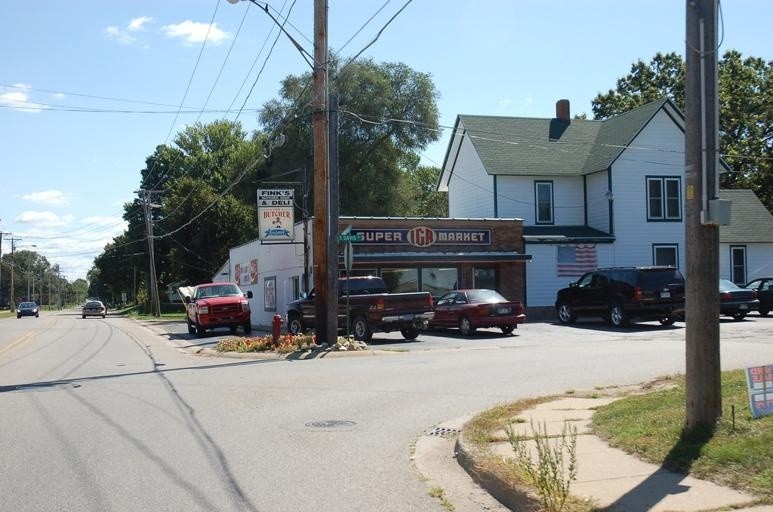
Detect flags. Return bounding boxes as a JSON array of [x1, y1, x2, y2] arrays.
[[557, 246, 598, 277]]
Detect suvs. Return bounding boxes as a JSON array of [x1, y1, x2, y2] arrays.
[[555, 265, 686, 328]]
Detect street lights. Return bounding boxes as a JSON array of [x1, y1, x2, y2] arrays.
[[228, 0, 328, 346], [11, 245, 37, 312]]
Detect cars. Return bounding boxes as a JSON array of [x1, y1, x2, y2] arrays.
[[16, 302, 39, 318], [82, 301, 106, 318], [421, 289, 526, 336], [719, 278, 773, 320]]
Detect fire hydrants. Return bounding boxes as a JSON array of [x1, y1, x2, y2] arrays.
[[272, 313, 284, 348]]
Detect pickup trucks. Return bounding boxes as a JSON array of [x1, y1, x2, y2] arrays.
[[185, 282, 252, 338], [285, 276, 435, 342]]
